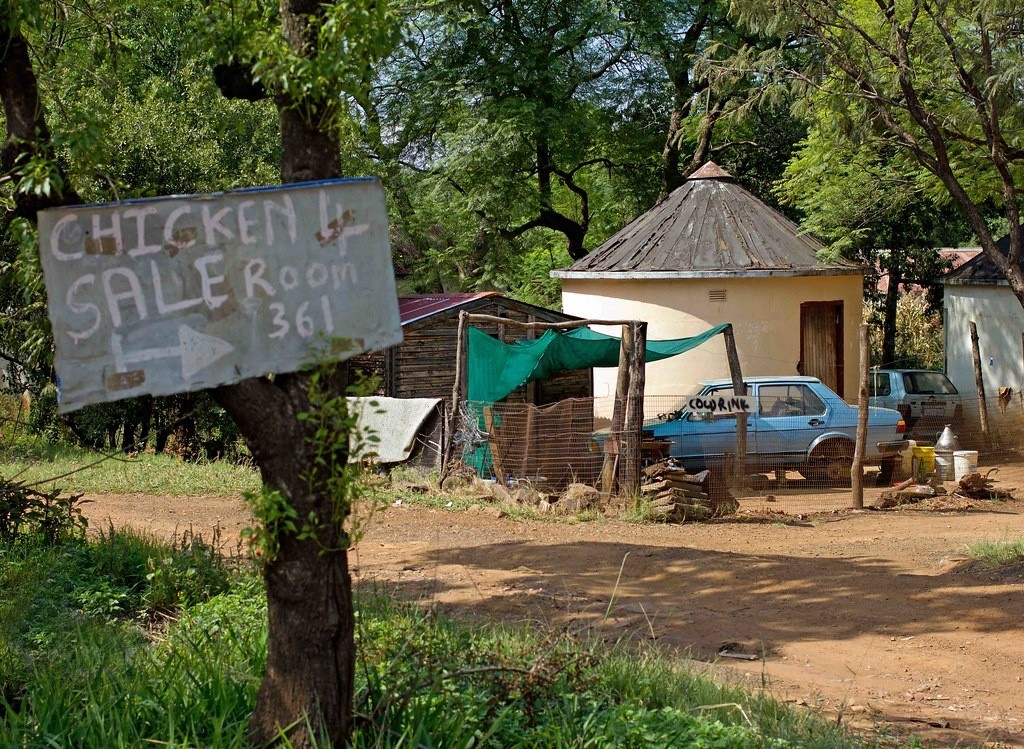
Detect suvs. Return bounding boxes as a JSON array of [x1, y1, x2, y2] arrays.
[[870, 369, 963, 446]]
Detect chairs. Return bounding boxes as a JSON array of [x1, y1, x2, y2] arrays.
[[770, 398, 788, 418]]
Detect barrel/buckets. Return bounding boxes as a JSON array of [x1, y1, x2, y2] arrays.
[[912, 447, 935, 485], [953, 450, 978, 483], [899, 440, 917, 475]]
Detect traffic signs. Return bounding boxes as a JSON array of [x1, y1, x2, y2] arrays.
[[38, 177, 403, 416]]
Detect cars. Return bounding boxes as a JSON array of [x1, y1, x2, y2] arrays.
[[586, 376, 909, 484]]
